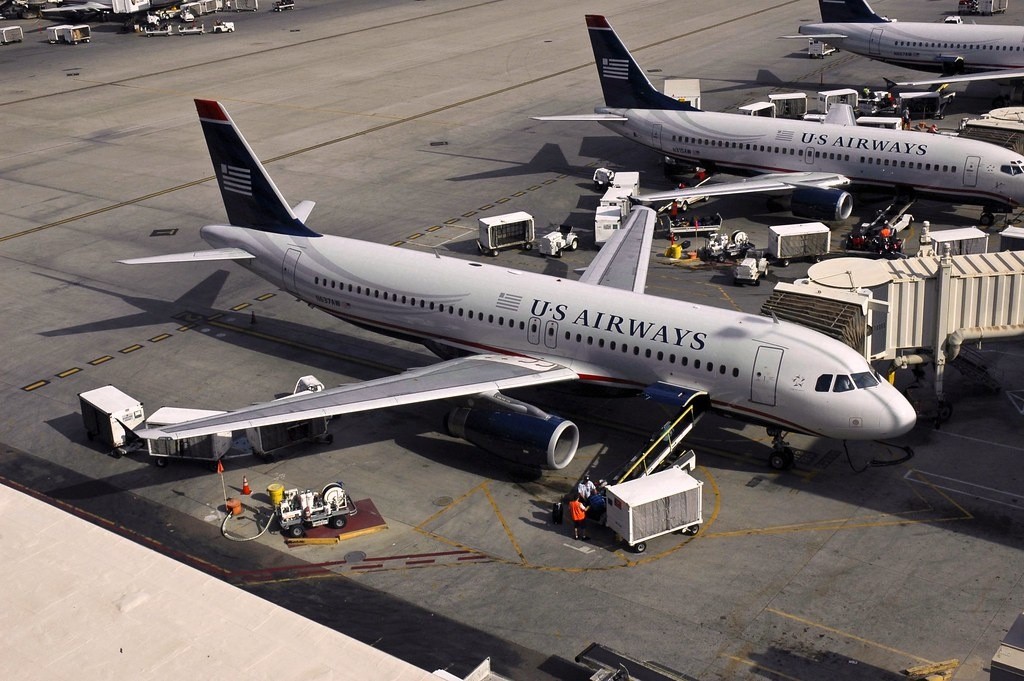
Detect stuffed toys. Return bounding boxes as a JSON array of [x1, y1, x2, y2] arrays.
[[690, 166, 705, 180]]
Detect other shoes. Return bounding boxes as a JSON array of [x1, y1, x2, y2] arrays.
[[582, 536, 591, 540], [575, 536, 580, 540]]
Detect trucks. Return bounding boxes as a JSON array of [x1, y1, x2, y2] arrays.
[[538, 226, 579, 258], [731, 257, 770, 286]]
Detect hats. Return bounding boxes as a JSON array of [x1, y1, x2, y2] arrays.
[[584, 476, 590, 481], [696, 167, 699, 171]]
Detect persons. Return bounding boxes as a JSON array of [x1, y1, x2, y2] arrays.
[[671, 200, 678, 221], [578, 476, 597, 505], [928, 124, 939, 135], [862, 86, 870, 99], [883, 15, 888, 21], [568, 494, 591, 541], [903, 107, 910, 130]]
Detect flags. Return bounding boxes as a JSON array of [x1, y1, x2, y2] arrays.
[[217, 461, 224, 474]]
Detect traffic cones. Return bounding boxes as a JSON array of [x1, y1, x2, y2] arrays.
[[240, 476, 252, 495]]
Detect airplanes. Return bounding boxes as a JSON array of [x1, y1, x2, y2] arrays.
[[113, 98, 916, 545], [527, 14, 1024, 206], [775, 0, 1024, 91]]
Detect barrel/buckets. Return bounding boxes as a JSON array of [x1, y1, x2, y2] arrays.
[[267, 484, 284, 508], [671, 244, 682, 259], [697, 171, 704, 179]]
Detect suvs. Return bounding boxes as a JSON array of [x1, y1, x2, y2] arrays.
[[272, 0, 295, 12], [213, 21, 235, 32]]
